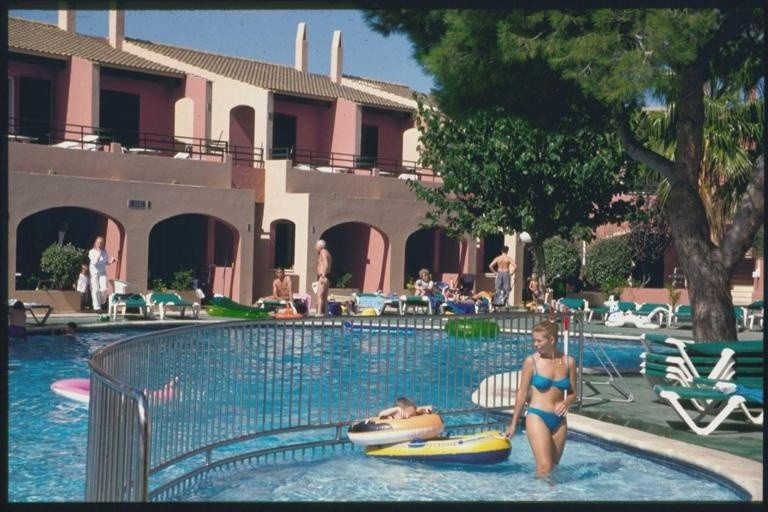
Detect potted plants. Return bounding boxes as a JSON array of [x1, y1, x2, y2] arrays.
[[164, 265, 203, 310]]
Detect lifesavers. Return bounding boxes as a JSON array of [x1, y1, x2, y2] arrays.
[[348, 412, 443, 445], [366, 430, 512, 466], [51, 376, 176, 406], [445, 319, 501, 338], [207, 305, 266, 318], [273, 313, 303, 319]]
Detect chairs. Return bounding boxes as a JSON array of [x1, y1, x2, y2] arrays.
[[546, 297, 764, 434], [106, 277, 204, 322], [256, 286, 496, 314]]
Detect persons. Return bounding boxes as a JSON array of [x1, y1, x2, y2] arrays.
[[411, 267, 551, 313], [487, 245, 517, 308], [312, 239, 332, 317], [88, 236, 119, 313], [379, 397, 433, 420], [250, 265, 294, 309], [504, 320, 582, 480]]
